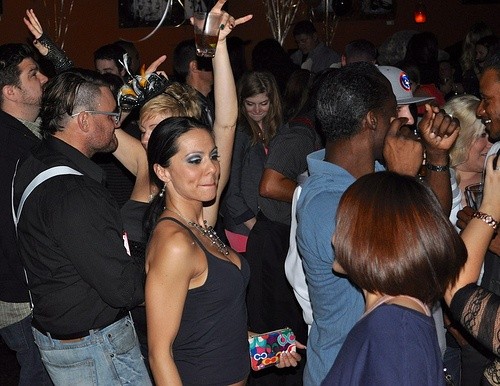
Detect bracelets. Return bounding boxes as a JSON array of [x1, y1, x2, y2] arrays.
[[426, 162, 448, 171], [474, 211, 498, 230]]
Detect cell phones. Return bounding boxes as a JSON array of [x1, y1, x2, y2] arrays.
[[248, 328, 298, 372]]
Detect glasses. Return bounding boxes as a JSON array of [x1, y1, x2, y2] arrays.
[[69, 106, 121, 125]]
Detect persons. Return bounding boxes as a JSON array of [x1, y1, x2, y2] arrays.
[[0, 0, 500, 386]]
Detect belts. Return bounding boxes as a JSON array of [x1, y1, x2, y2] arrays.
[[31, 317, 109, 340]]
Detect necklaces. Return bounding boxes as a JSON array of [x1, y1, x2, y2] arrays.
[[166, 207, 230, 256]]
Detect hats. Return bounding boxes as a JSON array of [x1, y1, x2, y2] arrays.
[[374, 64, 436, 105]]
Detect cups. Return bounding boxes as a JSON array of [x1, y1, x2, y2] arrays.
[[465, 182, 484, 210], [193, 11, 223, 58]]
